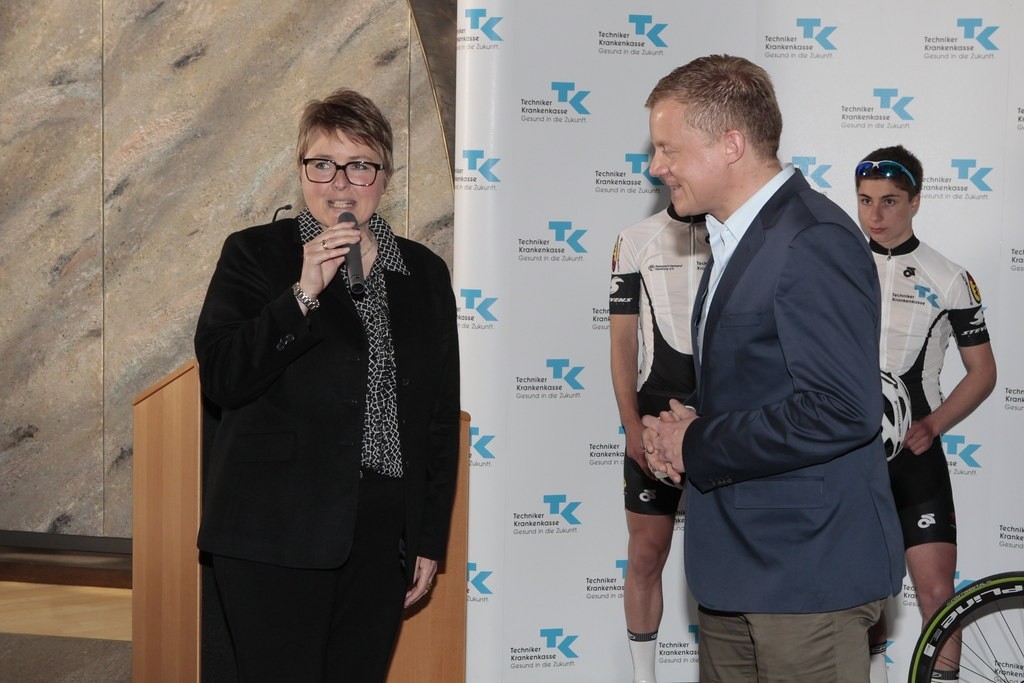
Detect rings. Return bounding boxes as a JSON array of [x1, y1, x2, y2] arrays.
[[426, 583, 431, 590], [322, 240, 328, 250]]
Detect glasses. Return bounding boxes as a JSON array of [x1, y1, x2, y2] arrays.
[[301, 157, 385, 187], [856, 160, 918, 193]]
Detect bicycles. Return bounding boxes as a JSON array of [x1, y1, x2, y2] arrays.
[[908, 571, 1024, 683]]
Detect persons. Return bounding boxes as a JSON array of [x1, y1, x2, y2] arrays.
[[855, 145, 998, 683], [193, 88, 460, 683], [609, 199, 713, 683], [643, 57, 908, 683]]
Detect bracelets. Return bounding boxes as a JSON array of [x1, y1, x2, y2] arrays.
[[292, 281, 319, 311]]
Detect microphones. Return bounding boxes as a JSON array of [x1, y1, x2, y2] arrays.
[[337, 212, 365, 294], [272, 204, 292, 222]]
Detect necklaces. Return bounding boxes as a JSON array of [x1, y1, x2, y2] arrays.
[[361, 229, 376, 257]]
